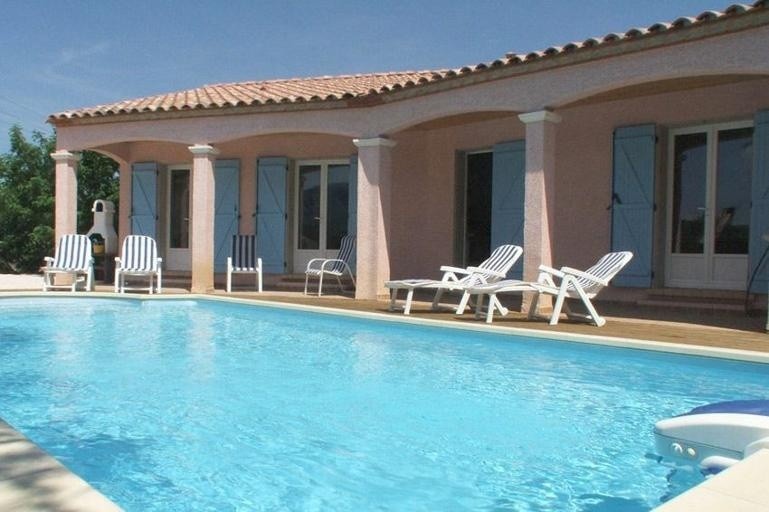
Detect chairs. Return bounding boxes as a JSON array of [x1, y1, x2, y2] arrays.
[[227, 235, 262, 293], [384, 244, 634, 327], [40, 234, 163, 294], [304, 230, 356, 299]]
